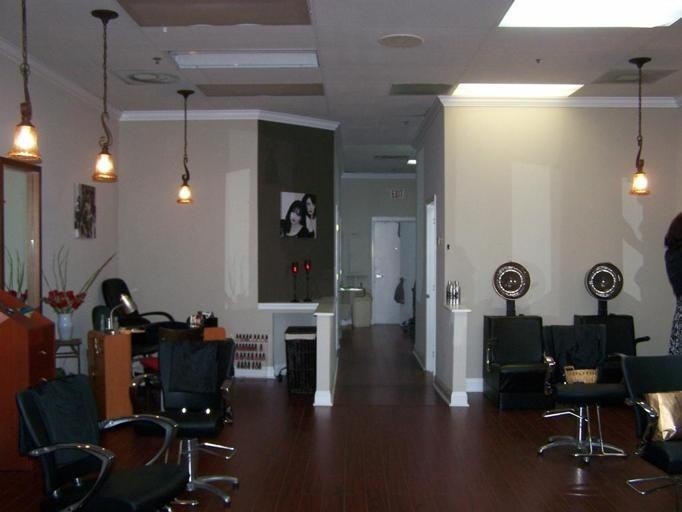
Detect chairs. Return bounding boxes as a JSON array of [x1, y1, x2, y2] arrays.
[[483, 315, 682, 495]]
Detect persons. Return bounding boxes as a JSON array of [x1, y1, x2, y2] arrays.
[[78, 200, 96, 237], [665, 211, 682, 356], [302, 193, 317, 239], [280, 200, 308, 240]]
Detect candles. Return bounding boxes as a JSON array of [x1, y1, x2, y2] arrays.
[[305, 260, 312, 271], [290, 261, 298, 273]]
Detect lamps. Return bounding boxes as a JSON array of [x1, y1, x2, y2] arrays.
[[628, 57, 652, 195], [177, 89, 195, 203], [8, 0, 40, 161], [91, 9, 120, 182]]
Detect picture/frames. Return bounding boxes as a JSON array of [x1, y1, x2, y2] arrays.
[[74, 183, 96, 237]]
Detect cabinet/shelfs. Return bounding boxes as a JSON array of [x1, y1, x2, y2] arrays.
[[0, 289, 55, 471]]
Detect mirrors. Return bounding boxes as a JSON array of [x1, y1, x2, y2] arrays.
[[0, 157, 42, 316]]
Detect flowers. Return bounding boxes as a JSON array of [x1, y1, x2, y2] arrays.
[[0, 246, 29, 310], [41, 245, 117, 313]]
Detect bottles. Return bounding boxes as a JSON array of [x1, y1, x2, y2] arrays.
[[447, 280, 461, 304]]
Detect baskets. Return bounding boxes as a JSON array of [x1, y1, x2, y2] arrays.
[[564, 366, 599, 385]]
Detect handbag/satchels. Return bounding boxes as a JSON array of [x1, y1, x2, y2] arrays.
[[643, 391, 682, 442]]
